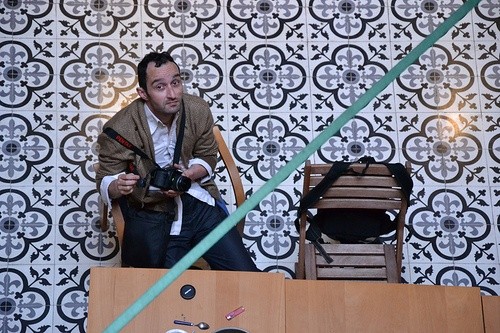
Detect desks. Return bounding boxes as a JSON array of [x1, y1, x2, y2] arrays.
[[86, 268, 500, 333]]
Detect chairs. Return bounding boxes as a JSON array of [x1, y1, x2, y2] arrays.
[[294, 157, 412, 283], [93, 126, 247, 268]]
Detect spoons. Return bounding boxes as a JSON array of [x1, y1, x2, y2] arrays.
[[174, 320, 209, 330]]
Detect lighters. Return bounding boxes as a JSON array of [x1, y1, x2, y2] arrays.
[[225, 305, 245, 320]]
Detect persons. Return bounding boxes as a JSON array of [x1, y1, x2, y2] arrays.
[[95, 51, 263, 272]]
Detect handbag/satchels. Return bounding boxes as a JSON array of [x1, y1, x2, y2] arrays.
[[122, 206, 174, 269]]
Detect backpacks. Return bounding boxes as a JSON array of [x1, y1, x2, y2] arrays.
[[294, 157, 413, 264]]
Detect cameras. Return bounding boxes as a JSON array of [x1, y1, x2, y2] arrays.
[[150, 167, 191, 192]]
